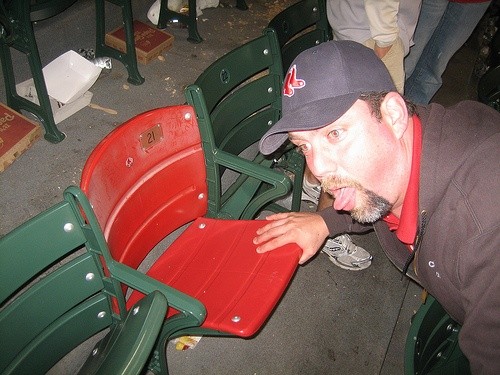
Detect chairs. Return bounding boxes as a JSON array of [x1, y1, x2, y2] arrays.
[[0, 0, 500, 375]]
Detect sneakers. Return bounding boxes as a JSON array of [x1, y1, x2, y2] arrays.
[[300, 177, 322, 204], [320, 232, 372, 271]]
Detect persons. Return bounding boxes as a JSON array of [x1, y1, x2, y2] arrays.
[[319, 0, 493, 270], [253, 39, 500, 375]]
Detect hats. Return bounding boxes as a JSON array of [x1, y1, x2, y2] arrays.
[[259, 39, 397, 156]]
